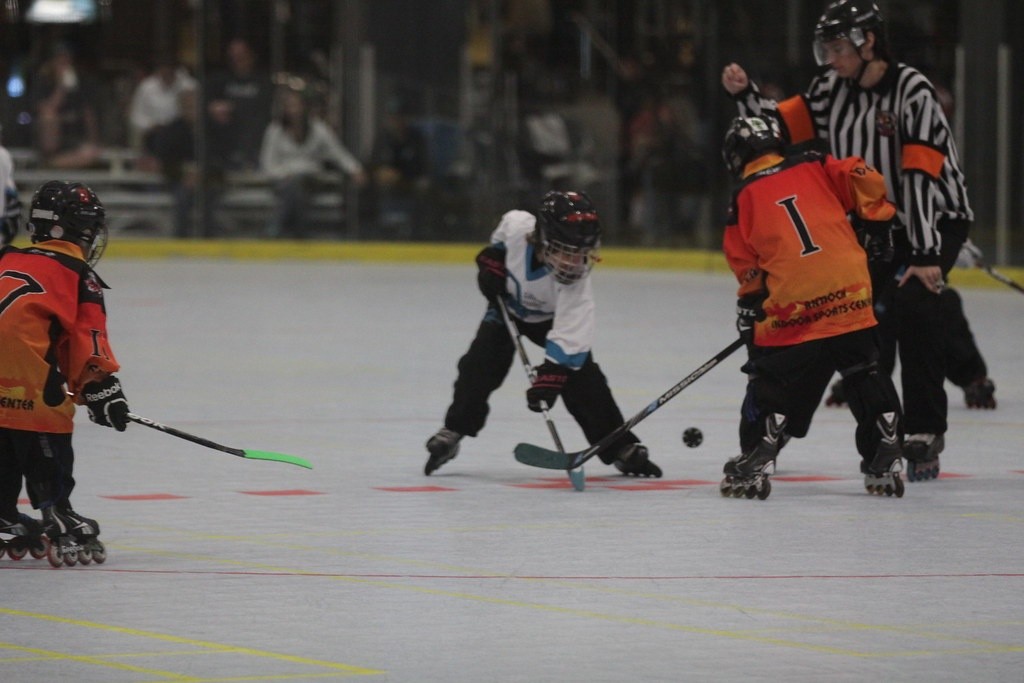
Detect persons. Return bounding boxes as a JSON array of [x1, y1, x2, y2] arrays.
[[723, 114, 907, 477], [0, 34, 982, 270], [0, 179, 131, 545], [426, 189, 648, 471], [831, 287, 999, 407], [723, 0, 974, 463]]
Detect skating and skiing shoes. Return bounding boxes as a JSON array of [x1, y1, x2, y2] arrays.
[[965, 377, 996, 409], [0, 513, 50, 561], [720, 413, 792, 500], [41, 500, 106, 568], [827, 381, 847, 407], [901, 433, 943, 480], [615, 444, 662, 478], [425, 427, 463, 475], [861, 412, 906, 497]]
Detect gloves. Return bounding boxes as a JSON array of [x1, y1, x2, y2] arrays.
[[526, 359, 575, 413], [736, 291, 769, 348], [83, 376, 131, 432], [0, 216, 20, 245], [865, 215, 909, 279], [476, 243, 507, 302]]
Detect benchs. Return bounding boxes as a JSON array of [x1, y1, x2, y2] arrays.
[[16, 148, 344, 237]]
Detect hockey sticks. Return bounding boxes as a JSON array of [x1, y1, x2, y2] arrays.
[[498, 291, 586, 493], [513, 337, 746, 471], [965, 245, 1024, 294], [65, 389, 315, 471]]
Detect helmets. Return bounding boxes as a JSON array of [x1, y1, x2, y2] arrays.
[[26, 180, 108, 269], [535, 191, 602, 285], [723, 117, 786, 174], [815, 0, 884, 43]]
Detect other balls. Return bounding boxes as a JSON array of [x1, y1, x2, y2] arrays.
[[682, 427, 702, 448]]
